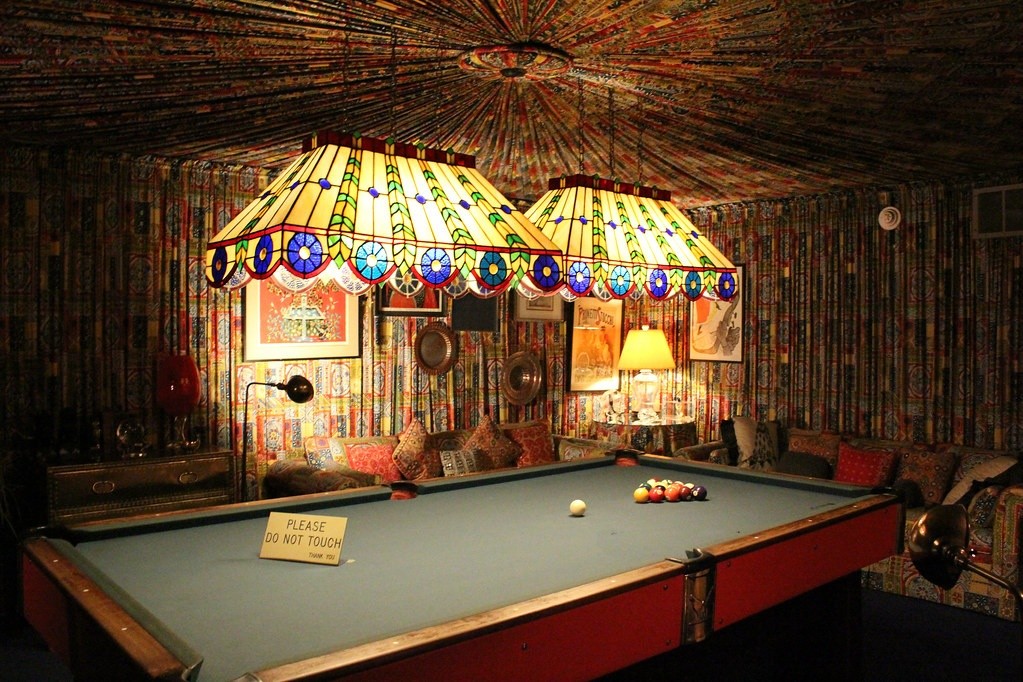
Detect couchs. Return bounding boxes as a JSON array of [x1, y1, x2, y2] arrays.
[[260, 426, 623, 497], [673, 428, 1023, 621]]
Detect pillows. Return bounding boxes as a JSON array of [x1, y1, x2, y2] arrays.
[[708, 416, 1019, 506], [304, 414, 556, 480]]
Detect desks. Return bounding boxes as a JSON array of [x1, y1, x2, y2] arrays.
[[592, 412, 694, 456], [19, 448, 906, 682]]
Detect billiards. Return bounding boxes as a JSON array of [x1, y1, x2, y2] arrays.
[[633, 477, 708, 503], [569, 499, 586, 517]]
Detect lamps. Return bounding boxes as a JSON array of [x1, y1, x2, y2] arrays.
[[617, 324, 677, 420], [242, 375, 314, 500], [909, 501, 1023, 604]]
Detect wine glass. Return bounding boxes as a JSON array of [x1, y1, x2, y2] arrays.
[[155, 356, 200, 453]]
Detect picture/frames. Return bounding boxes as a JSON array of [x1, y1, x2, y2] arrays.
[[688, 264, 748, 362], [241, 277, 361, 361], [566, 295, 625, 392], [374, 270, 445, 316], [447, 291, 499, 334], [508, 281, 565, 323]]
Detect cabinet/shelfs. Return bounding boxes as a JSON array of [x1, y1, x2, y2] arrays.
[[35, 449, 238, 527]]
